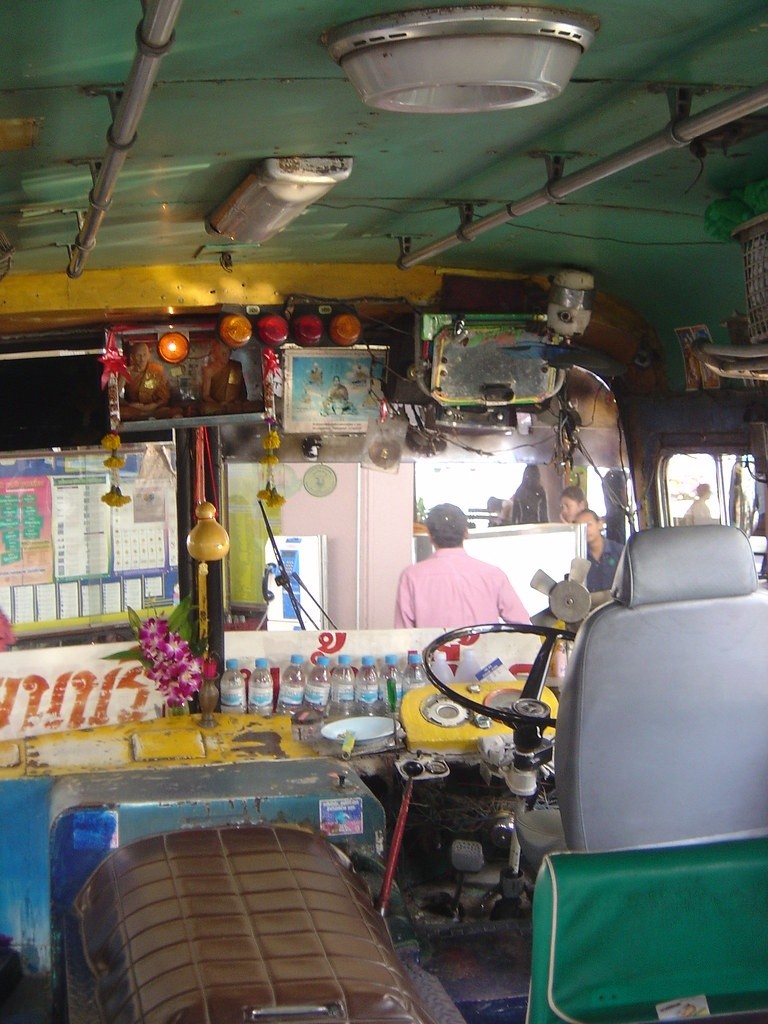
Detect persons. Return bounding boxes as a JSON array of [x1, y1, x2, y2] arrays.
[[560, 486, 588, 523], [393, 503, 531, 629], [198, 340, 263, 415], [118, 342, 183, 420], [513, 465, 548, 524], [684, 484, 711, 526], [602, 470, 626, 545], [753, 476, 768, 579], [323, 375, 349, 414], [311, 362, 323, 381], [575, 509, 623, 593], [488, 497, 513, 526]]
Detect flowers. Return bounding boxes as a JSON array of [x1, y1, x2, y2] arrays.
[[100, 592, 210, 708]]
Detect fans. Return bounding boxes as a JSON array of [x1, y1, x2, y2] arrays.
[[528, 557, 616, 632]]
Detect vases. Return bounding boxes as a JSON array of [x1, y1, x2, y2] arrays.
[[165, 699, 190, 717]]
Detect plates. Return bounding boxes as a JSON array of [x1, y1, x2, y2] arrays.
[[321, 717, 400, 744]]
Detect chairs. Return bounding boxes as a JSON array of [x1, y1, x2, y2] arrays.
[[510, 524, 768, 1024]]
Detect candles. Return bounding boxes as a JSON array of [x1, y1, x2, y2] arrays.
[[203, 658, 217, 678]]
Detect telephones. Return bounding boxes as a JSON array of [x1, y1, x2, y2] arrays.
[[261, 563, 277, 601]]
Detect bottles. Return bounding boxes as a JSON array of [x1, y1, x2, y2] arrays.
[[276, 654, 304, 712], [378, 654, 402, 718], [304, 656, 331, 715], [248, 658, 273, 715], [428, 653, 454, 686], [221, 659, 246, 715], [330, 654, 355, 716], [418, 499, 424, 517], [454, 649, 482, 684], [401, 654, 427, 699], [354, 656, 378, 717]]
